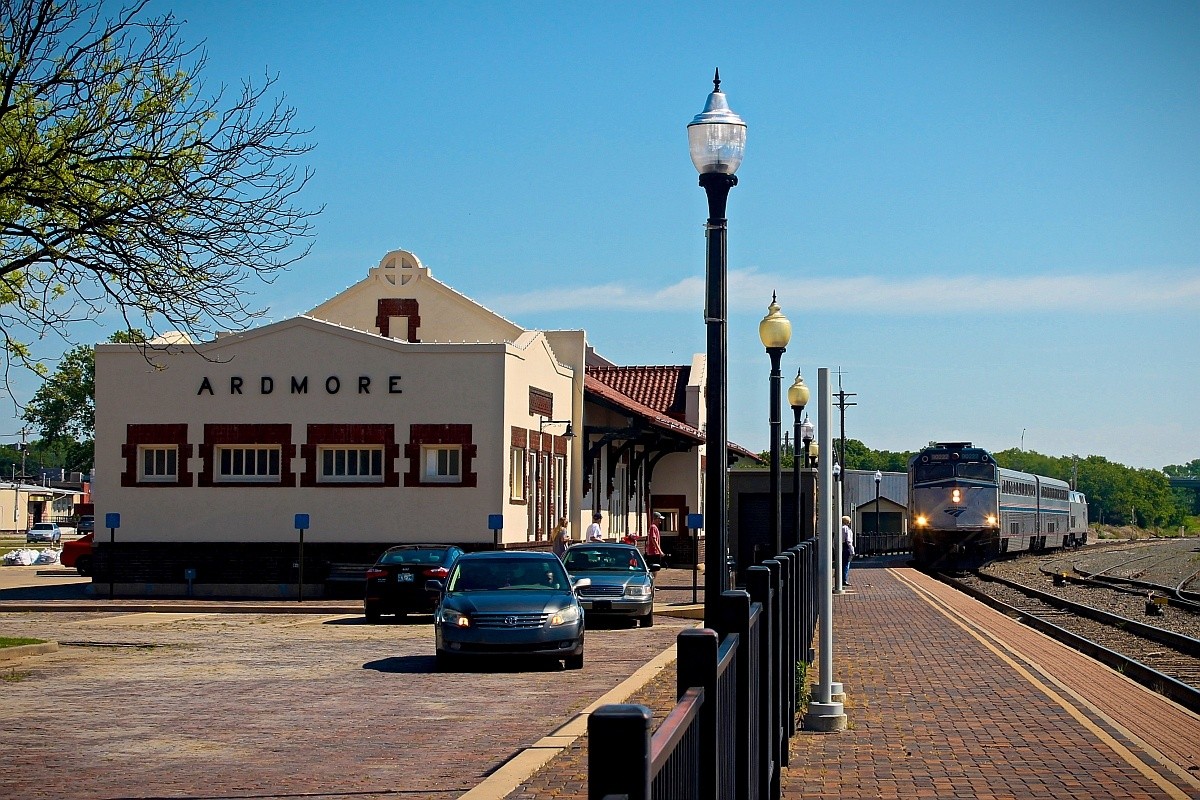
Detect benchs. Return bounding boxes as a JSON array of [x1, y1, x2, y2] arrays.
[[323, 560, 375, 601]]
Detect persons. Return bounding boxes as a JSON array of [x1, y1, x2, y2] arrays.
[[586, 513, 606, 543], [832, 516, 856, 586], [550, 517, 573, 558], [646, 512, 666, 591], [620, 534, 640, 548]]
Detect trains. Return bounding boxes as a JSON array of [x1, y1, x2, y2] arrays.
[[905, 439, 1089, 566]]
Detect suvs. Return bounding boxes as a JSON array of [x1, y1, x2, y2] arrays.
[[77, 515, 95, 534]]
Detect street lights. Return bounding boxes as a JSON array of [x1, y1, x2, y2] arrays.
[[874, 470, 882, 534], [833, 463, 840, 483], [788, 367, 819, 545], [686, 66, 748, 632], [758, 288, 792, 554]]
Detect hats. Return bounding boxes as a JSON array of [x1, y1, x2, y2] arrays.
[[653, 513, 665, 520], [593, 514, 602, 520]]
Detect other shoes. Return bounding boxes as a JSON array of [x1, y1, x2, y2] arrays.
[[843, 581, 852, 586], [655, 588, 660, 591]]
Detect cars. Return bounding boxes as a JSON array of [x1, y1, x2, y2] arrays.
[[424, 549, 592, 672], [559, 542, 662, 628], [60, 533, 94, 577], [26, 522, 61, 545], [364, 544, 467, 620]]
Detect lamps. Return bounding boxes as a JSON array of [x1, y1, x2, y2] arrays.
[[540, 416, 578, 441]]
